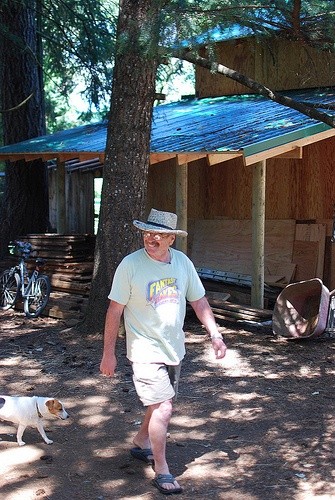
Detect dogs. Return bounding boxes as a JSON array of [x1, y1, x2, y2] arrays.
[[0, 395, 69, 446]]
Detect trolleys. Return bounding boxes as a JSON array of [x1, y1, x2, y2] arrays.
[[271, 277, 335, 340]]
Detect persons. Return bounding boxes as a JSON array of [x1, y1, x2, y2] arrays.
[[100, 209, 227, 494]]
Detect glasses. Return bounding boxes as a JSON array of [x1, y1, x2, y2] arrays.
[[141, 231, 169, 240]]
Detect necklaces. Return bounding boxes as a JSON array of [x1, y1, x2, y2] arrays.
[[145, 248, 172, 265]]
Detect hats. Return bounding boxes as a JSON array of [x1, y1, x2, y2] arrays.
[[133, 208, 188, 237]]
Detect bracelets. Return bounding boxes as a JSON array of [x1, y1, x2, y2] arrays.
[[211, 333, 223, 339]]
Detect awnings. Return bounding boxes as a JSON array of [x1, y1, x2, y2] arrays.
[[1, 87, 335, 166]]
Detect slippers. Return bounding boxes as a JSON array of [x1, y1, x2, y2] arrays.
[[152, 474, 183, 494], [132, 447, 155, 465]]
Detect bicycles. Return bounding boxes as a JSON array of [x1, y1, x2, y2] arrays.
[[0, 241, 50, 318]]
